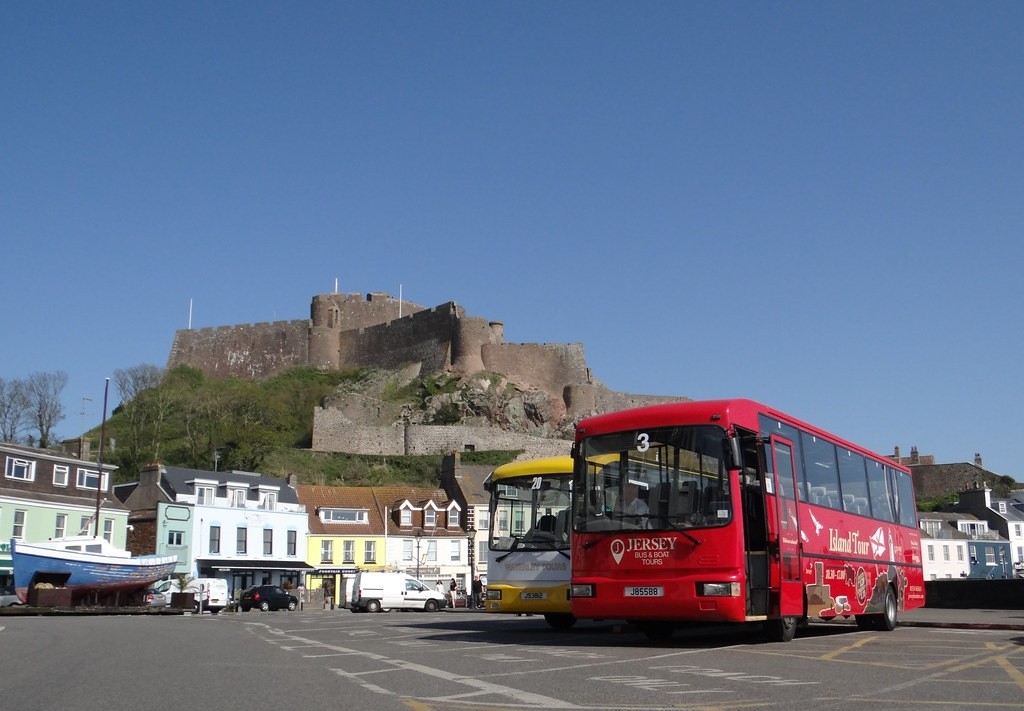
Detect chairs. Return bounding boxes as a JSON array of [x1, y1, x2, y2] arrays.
[[538, 513, 562, 531], [614, 472, 868, 527]]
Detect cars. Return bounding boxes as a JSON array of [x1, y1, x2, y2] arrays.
[[142, 588, 167, 608], [240, 585, 299, 613]]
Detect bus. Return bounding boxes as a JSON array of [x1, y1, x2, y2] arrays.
[[483, 452, 728, 629], [567, 398, 926, 641]]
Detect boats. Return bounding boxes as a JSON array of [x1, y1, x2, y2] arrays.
[[10, 377, 179, 605]]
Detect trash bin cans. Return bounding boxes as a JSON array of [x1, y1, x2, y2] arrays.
[[298, 602, 304, 611]]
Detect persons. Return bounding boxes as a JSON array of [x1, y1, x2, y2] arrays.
[[472, 575, 484, 609], [613, 483, 654, 529], [297, 582, 305, 602], [435, 580, 445, 597], [449, 578, 457, 608]]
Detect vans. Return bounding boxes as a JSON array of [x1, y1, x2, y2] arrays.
[[350, 571, 445, 612], [156, 578, 229, 614]]
